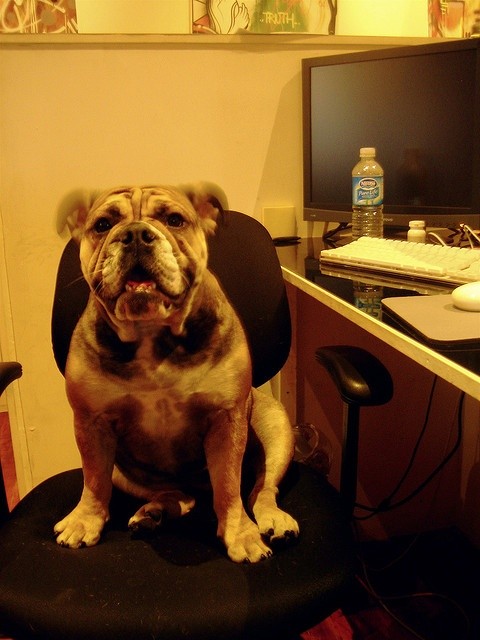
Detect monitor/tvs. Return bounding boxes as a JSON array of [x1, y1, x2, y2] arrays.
[[302, 36, 480, 244]]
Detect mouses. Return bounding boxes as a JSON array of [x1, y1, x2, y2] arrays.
[[452, 281, 480, 311]]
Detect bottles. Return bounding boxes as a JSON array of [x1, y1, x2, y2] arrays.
[[351, 147, 383, 240], [407, 220, 426, 242], [354, 281, 383, 325]]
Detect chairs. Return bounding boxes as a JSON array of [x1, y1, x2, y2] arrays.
[[0, 210, 347, 636]]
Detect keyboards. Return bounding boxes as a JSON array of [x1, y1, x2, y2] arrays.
[[318, 265, 455, 295], [319, 234, 480, 285]]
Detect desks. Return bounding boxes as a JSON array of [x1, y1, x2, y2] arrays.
[[273, 235, 479, 638]]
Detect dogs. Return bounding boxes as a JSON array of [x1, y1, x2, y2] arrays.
[[48, 179, 303, 565]]
